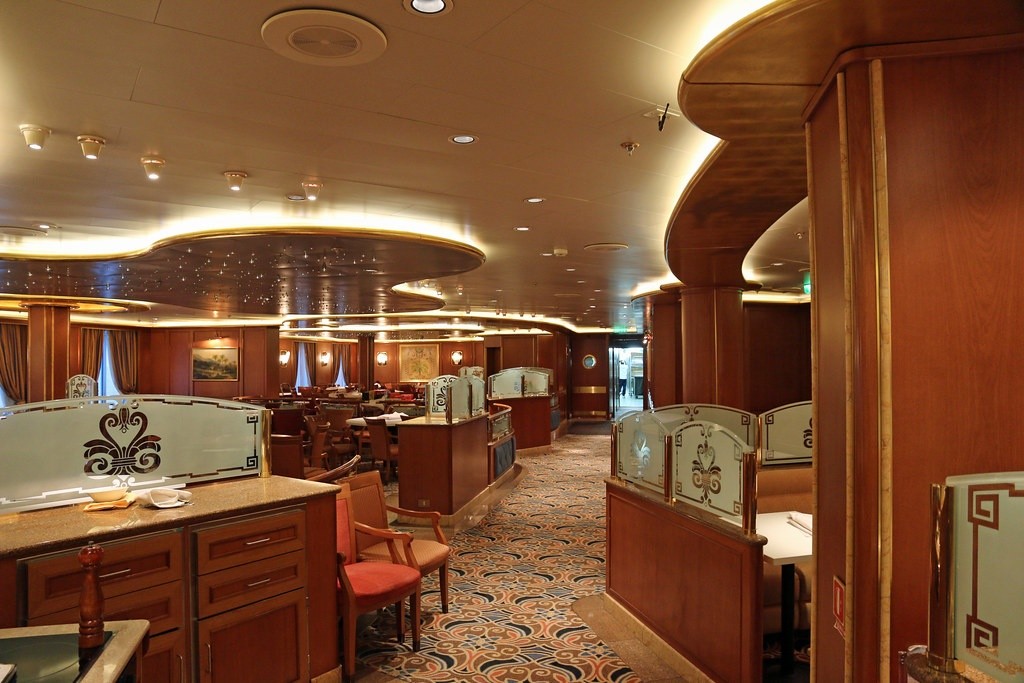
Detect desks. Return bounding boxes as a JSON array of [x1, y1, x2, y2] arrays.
[[725, 509, 813, 683], [344, 415, 402, 426]]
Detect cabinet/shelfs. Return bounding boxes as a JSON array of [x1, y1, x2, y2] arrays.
[[2, 476, 342, 683]]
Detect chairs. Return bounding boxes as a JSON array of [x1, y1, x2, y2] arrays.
[[234, 382, 453, 676]]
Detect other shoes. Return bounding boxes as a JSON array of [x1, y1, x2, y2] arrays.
[[621, 393, 625, 398]]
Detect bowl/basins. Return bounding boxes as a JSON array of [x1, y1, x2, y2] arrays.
[[86, 486, 129, 502]]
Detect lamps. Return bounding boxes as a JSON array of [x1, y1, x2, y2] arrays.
[[20, 125, 52, 151], [301, 181, 324, 201], [76, 135, 104, 160], [140, 157, 167, 179], [224, 170, 247, 191]]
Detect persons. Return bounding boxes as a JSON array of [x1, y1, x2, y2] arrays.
[[619, 360, 628, 397]]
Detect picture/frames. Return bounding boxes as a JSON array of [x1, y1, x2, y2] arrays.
[[193, 347, 239, 382]]
